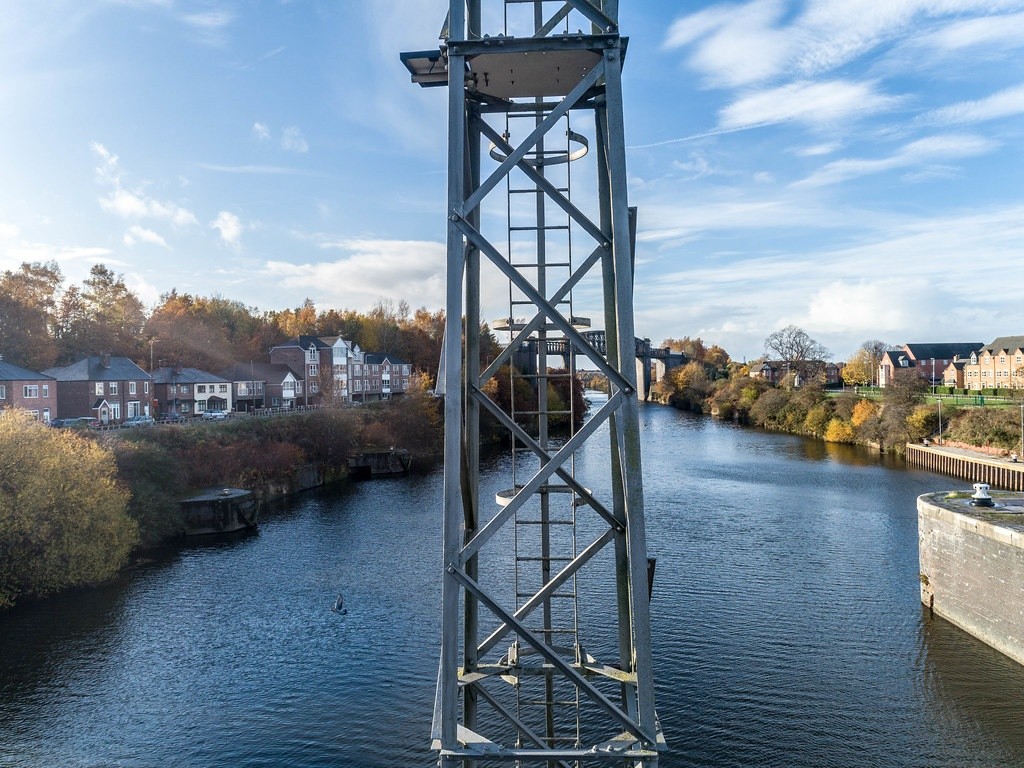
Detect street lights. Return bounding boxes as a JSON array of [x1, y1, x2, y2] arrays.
[[1019, 404, 1024, 462], [787, 363, 791, 371], [931, 357, 934, 396], [936, 398, 943, 444], [870, 361, 873, 393]]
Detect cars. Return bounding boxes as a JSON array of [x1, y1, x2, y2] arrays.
[[123, 416, 155, 428], [156, 413, 185, 424], [200, 409, 225, 420]]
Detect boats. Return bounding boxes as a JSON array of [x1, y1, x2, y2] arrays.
[[350, 448, 416, 480], [177, 485, 260, 543]]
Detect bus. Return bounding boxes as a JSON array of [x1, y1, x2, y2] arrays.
[[51, 418, 100, 434]]
